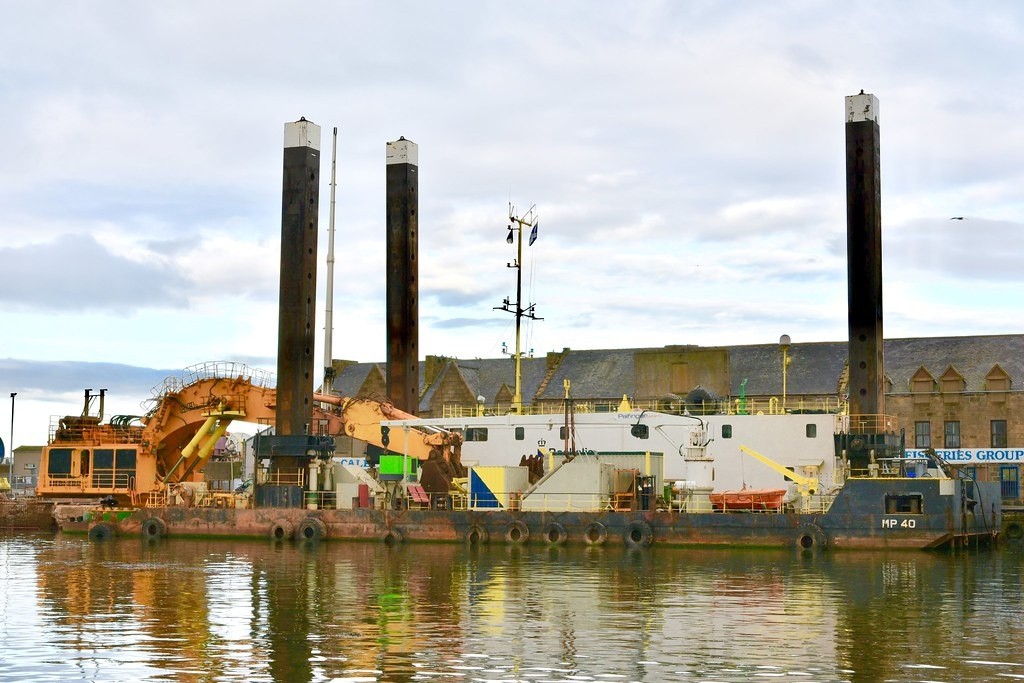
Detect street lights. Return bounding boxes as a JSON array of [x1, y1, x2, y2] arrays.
[[7, 392, 17, 499]]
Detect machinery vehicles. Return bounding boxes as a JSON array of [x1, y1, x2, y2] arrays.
[[35, 360, 468, 508]]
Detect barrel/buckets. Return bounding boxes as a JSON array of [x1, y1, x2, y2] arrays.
[[307, 490, 318, 510], [320, 490, 336, 510], [510, 491, 522, 511]]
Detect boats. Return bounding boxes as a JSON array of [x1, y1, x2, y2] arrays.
[[710, 486, 788, 510], [54, 200, 1004, 555]]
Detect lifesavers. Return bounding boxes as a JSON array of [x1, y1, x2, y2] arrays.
[[581, 521, 608, 546], [503, 519, 532, 547], [87, 522, 115, 537], [540, 521, 571, 548], [464, 523, 490, 545], [378, 527, 405, 547], [141, 516, 168, 537], [622, 519, 655, 548], [265, 516, 331, 542], [788, 520, 828, 554]]
